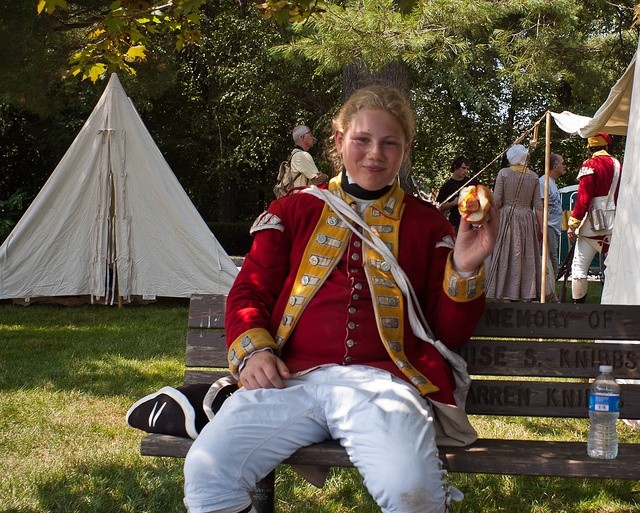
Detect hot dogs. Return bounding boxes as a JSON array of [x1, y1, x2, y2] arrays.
[[457, 184, 490, 224]]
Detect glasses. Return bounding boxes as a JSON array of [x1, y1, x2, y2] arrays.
[[302, 131, 314, 136]]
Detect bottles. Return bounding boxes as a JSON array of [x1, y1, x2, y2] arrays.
[[587, 365, 621, 460]]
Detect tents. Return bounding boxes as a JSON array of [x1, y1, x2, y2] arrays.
[[0, 73, 238, 307]]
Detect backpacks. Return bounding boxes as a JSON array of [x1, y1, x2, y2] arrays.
[[274, 151, 304, 198]]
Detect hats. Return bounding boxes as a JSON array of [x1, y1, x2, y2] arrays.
[[585, 134, 609, 148]]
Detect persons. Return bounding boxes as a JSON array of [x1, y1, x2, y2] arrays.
[[182, 84, 501, 513], [487, 144, 544, 303], [566, 132, 623, 303], [535, 152, 567, 304], [436, 156, 477, 234], [272, 124, 328, 199]]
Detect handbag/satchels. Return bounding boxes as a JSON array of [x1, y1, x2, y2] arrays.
[[388, 331, 480, 449], [574, 157, 621, 239]]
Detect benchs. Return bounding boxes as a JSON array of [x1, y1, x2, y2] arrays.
[[140, 293, 640, 513]]
